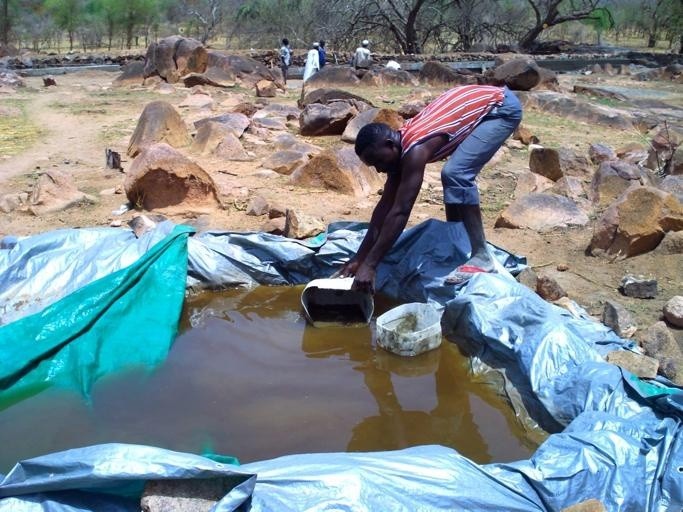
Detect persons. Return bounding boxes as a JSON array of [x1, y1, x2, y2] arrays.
[[279, 38, 292, 85], [351, 39, 372, 79], [316, 39, 326, 68], [330, 84, 522, 298], [302, 41, 319, 84]]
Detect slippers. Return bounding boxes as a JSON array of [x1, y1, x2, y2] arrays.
[[445, 265, 498, 286]]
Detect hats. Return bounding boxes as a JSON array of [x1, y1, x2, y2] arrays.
[[363, 40, 369, 45], [312, 43, 319, 48]]
